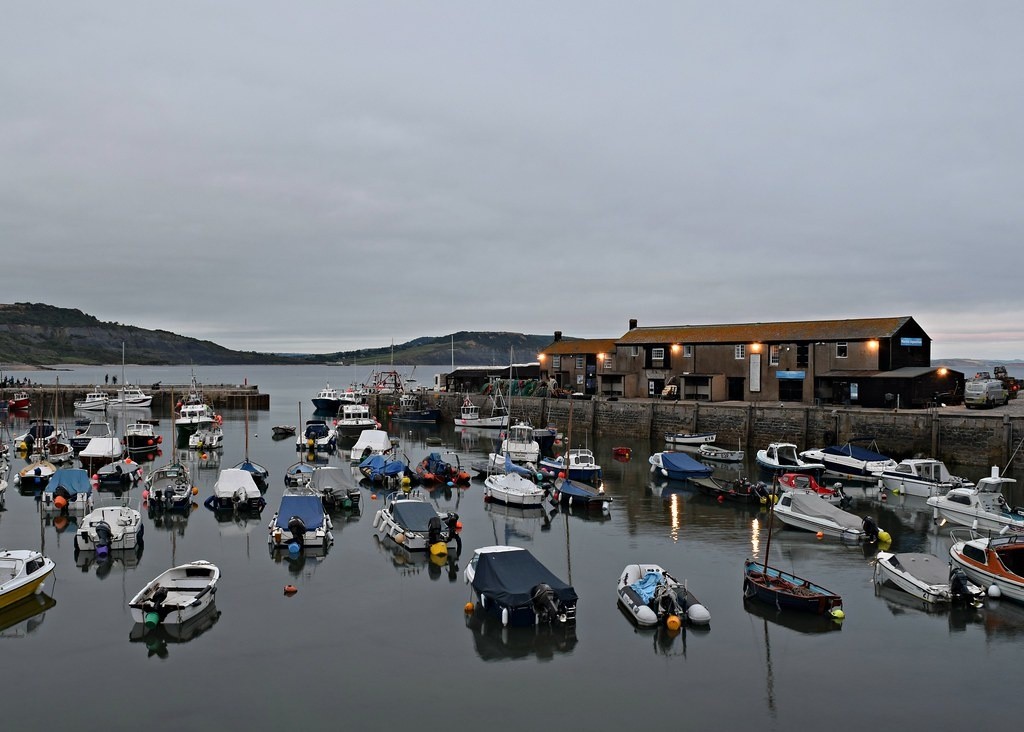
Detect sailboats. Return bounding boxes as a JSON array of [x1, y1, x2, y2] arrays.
[[741, 591, 844, 712], [740, 473, 844, 620], [484, 495, 551, 545]]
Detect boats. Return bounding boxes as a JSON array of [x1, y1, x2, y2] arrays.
[[665, 432, 719, 444], [611, 446, 632, 458], [646, 441, 1024, 541], [948, 527, 1024, 603], [874, 549, 985, 606], [873, 578, 986, 633], [0, 333, 614, 568], [373, 532, 460, 583], [74, 538, 144, 581], [462, 545, 579, 628], [616, 562, 712, 631], [0, 549, 55, 606], [0, 591, 58, 641], [268, 533, 333, 577], [128, 601, 221, 661], [128, 558, 222, 624]]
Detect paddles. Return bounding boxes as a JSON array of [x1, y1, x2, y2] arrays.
[[135, 582, 161, 605]]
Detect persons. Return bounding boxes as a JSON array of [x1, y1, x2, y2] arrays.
[[4, 376, 7, 382], [549, 375, 558, 390], [24, 377, 27, 382], [17, 379, 20, 385], [10, 375, 14, 383], [105, 373, 109, 383], [733, 477, 748, 493], [840, 493, 852, 509], [28, 378, 31, 385], [112, 376, 117, 384]]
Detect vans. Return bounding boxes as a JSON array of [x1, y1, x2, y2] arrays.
[[964, 379, 1009, 409]]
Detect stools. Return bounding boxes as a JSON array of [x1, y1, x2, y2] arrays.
[[815, 398, 823, 407]]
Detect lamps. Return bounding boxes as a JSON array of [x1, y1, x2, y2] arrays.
[[570, 355, 573, 358]]
